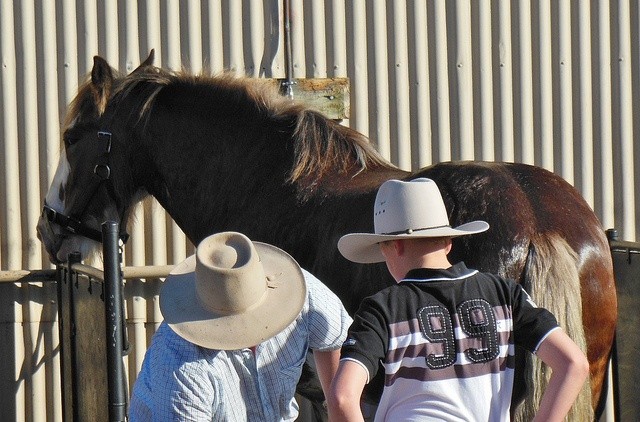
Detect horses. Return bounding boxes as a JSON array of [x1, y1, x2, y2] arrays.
[[34, 46, 619, 422]]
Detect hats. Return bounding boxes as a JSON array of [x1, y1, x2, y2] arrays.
[[159, 231, 306, 350], [338, 177, 491, 264]]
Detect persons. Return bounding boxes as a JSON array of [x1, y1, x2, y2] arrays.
[[127, 231, 354, 422], [328, 177, 589, 422]]
